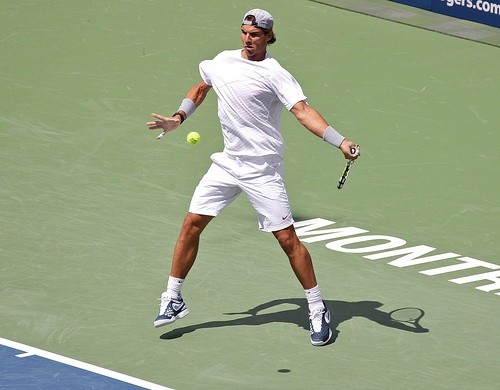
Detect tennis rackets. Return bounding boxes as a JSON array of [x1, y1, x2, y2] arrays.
[[337, 147, 355, 189]]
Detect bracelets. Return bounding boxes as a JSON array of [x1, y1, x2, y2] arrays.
[[172, 112, 184, 124]]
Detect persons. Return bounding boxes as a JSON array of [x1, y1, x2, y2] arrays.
[[146, 8, 360, 346]]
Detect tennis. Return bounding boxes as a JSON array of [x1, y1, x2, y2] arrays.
[[187, 131, 200, 145]]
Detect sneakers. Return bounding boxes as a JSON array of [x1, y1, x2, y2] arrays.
[[153, 293, 190, 328], [308, 300, 333, 347]]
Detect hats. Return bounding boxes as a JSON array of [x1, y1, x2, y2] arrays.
[[242, 8, 277, 45]]
